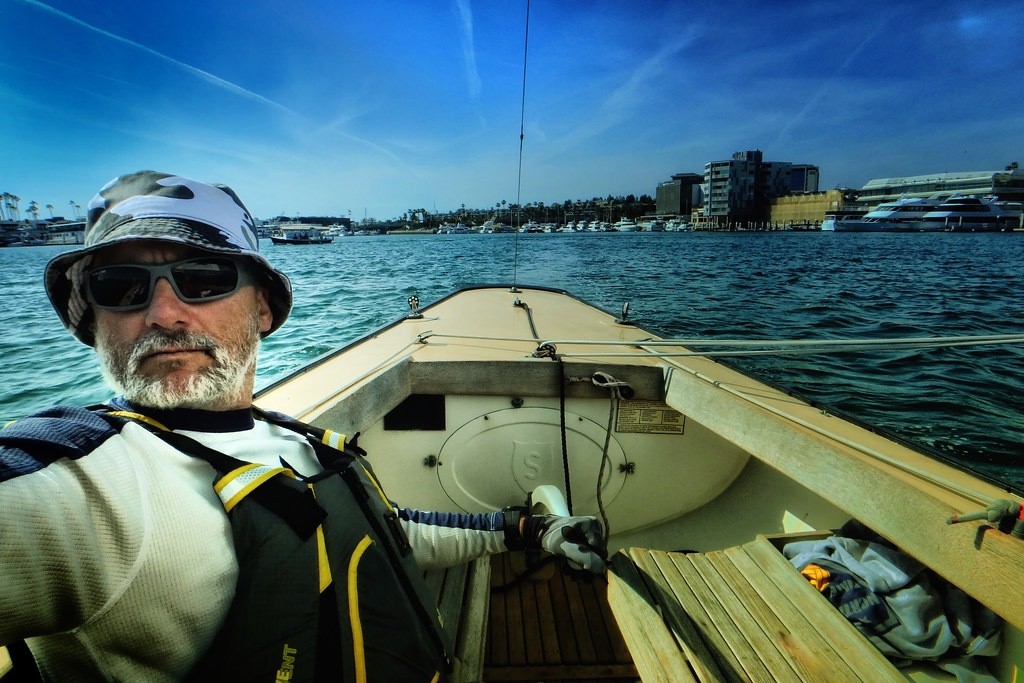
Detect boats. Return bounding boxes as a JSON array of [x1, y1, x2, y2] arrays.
[[820, 168, 1024, 234], [270, 225, 378, 245], [435, 214, 687, 235]]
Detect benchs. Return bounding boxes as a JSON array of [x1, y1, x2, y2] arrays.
[[418, 548, 490, 683], [597, 533, 909, 683]]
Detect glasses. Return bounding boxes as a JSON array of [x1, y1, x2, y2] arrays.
[[80, 255, 260, 312]]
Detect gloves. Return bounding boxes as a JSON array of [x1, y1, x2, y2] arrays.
[[522, 513, 607, 575]]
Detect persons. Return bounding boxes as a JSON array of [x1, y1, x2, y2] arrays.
[[0, 169, 604, 683]]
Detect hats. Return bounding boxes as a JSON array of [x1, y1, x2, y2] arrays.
[[43, 171, 292, 349]]
